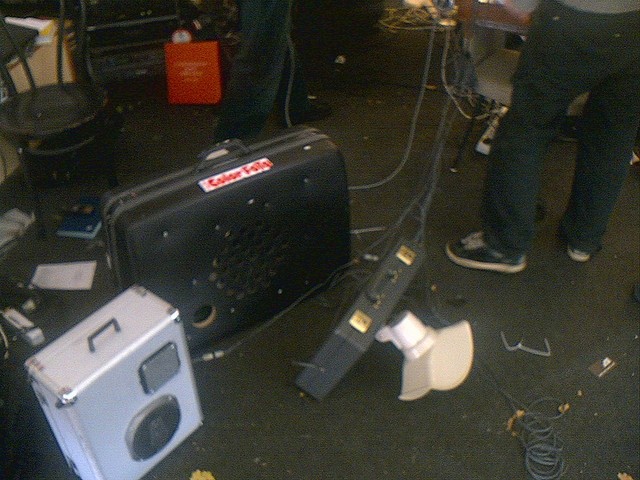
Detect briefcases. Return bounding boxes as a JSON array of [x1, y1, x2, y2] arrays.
[[294, 235, 428, 403], [99, 125, 352, 356]]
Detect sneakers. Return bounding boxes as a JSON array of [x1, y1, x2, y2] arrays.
[[444, 229, 527, 274]]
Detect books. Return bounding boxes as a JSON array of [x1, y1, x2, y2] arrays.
[[55, 198, 102, 239], [0, 207, 37, 255]]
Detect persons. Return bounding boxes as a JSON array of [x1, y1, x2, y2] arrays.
[[446, 0, 636, 275], [213, 4, 333, 145]]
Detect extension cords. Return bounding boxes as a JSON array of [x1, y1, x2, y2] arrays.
[[477, 106, 507, 156]]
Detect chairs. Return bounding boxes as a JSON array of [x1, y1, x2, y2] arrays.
[[1, 2, 120, 241]]
